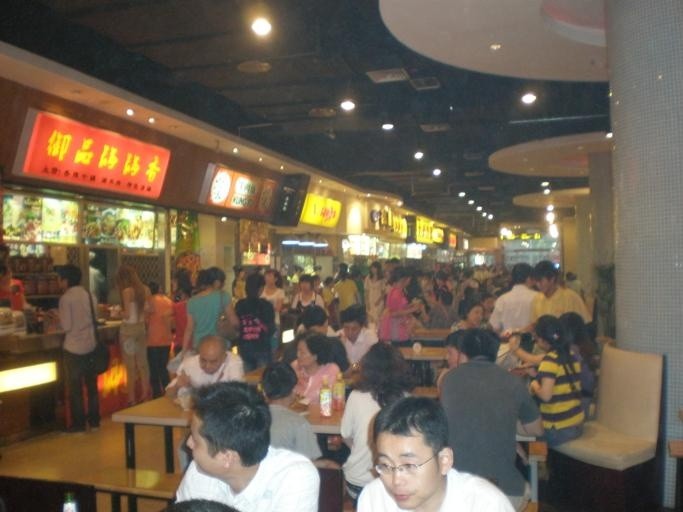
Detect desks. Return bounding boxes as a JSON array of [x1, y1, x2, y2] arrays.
[[111, 395, 549, 502], [413, 326, 451, 342], [396, 346, 450, 385], [247, 366, 440, 402], [1, 431, 183, 512]]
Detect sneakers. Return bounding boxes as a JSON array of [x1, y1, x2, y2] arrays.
[[60, 418, 100, 434]]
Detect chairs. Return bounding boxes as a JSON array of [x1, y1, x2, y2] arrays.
[[311, 457, 344, 512], [592, 336, 612, 364], [550, 341, 664, 512], [584, 294, 598, 344]]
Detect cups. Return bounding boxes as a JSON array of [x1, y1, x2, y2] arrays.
[[178, 394, 192, 412]]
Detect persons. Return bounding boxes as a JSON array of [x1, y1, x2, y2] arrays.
[[0, 262, 32, 313], [88, 250, 598, 512], [47, 263, 106, 436]]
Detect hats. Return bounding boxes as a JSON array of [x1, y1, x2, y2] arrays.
[[53, 264, 81, 281]]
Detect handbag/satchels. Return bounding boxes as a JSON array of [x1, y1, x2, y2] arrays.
[[495, 344, 518, 371], [93, 339, 110, 373], [379, 308, 414, 342], [217, 312, 240, 341]]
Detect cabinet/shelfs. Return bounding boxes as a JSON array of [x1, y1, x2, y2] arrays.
[[10, 270, 64, 299]]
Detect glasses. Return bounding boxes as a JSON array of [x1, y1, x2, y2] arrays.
[[374, 446, 442, 477]]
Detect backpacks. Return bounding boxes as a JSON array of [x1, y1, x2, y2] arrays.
[[238, 299, 266, 342]]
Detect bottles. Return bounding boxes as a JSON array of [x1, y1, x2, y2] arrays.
[[333, 370, 348, 409], [319, 374, 335, 418]]
